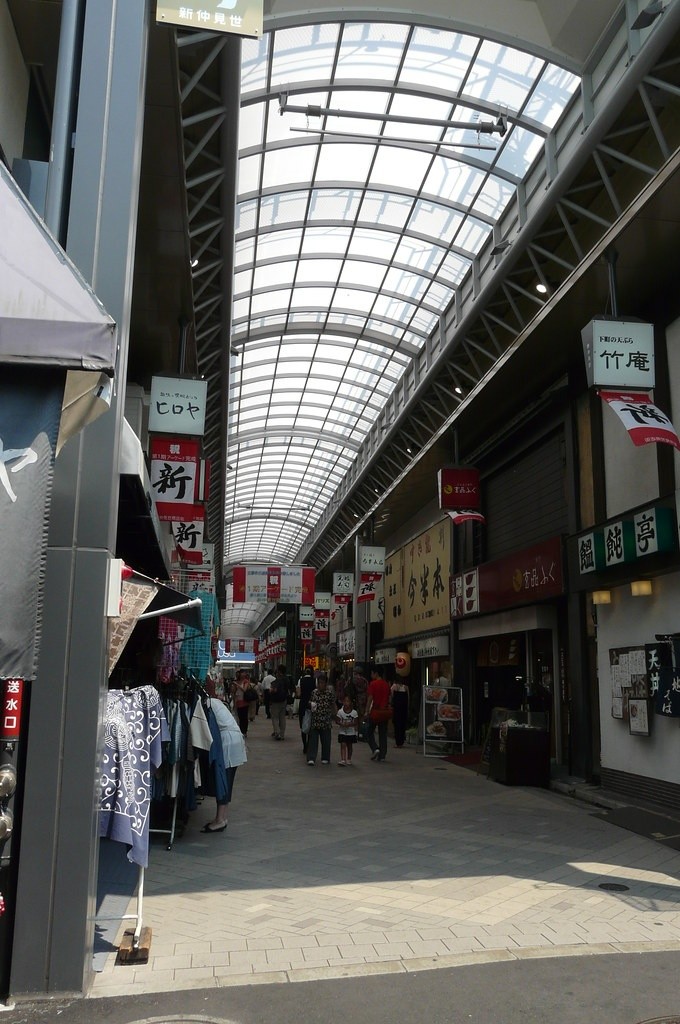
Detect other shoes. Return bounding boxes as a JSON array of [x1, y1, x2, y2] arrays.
[[243, 717, 287, 742], [322, 760, 328, 764], [338, 760, 347, 766], [307, 760, 315, 764], [346, 760, 353, 765], [372, 749, 385, 763]]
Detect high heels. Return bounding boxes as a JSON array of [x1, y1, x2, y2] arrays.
[[201, 823, 229, 832]]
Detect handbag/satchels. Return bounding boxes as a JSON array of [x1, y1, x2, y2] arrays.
[[370, 707, 393, 723], [244, 687, 258, 701]]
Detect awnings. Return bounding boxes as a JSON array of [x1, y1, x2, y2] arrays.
[[123, 569, 204, 633]]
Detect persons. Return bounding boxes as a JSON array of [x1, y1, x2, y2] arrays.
[[217, 664, 412, 765], [201, 697, 248, 833]]
